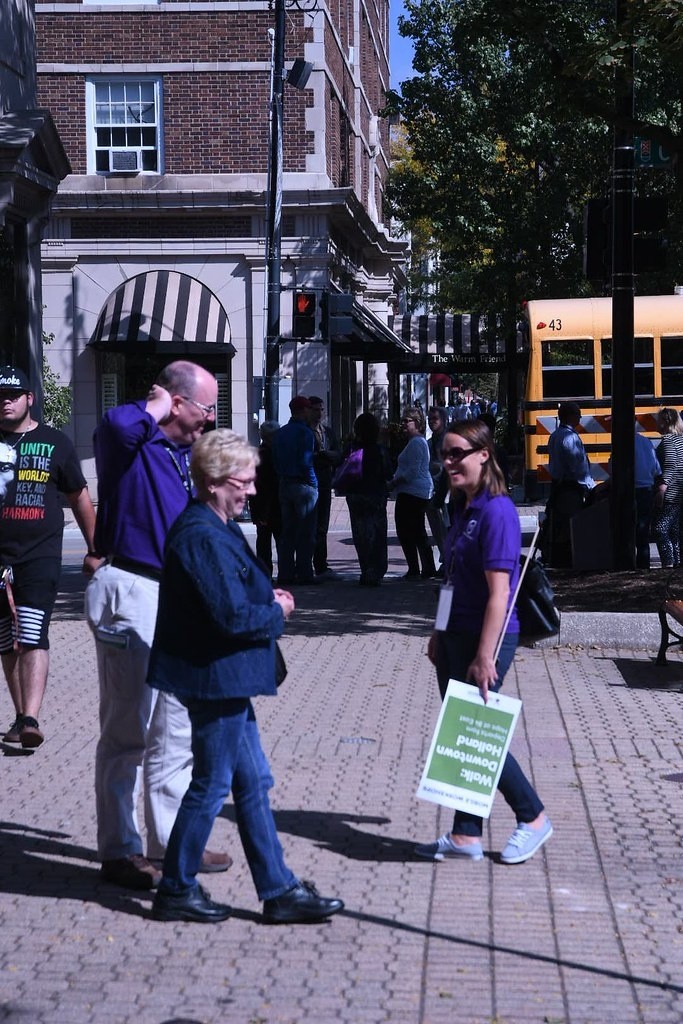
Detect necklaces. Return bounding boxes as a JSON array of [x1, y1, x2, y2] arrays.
[[0, 420, 32, 461]]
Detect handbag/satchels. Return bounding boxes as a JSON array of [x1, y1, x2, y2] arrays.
[[514, 551, 561, 648], [332, 443, 365, 487]]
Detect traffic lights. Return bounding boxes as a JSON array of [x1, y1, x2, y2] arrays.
[[292, 291, 316, 337]]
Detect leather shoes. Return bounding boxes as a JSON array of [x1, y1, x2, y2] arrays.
[[263, 880, 344, 923], [152, 881, 234, 921]]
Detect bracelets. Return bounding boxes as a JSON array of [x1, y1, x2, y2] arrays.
[[88, 551, 102, 559]]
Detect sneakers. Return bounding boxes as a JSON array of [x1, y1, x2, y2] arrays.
[[98, 852, 162, 889], [499, 816, 554, 863], [415, 831, 484, 862], [146, 846, 233, 872]]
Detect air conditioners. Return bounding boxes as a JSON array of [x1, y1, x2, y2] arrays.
[[108, 147, 143, 172]]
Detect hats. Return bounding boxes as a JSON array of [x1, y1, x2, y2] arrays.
[[261, 421, 280, 439], [0, 366, 31, 393], [289, 396, 311, 412]]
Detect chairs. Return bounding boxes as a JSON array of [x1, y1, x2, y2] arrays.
[[656, 568, 683, 666]]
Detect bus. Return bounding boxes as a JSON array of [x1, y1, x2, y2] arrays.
[[519, 293, 683, 483]]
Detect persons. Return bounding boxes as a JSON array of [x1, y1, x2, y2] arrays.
[[148, 428, 344, 927], [548, 403, 683, 571], [0, 365, 106, 747], [85, 361, 233, 889], [414, 418, 553, 863], [253, 390, 512, 585]]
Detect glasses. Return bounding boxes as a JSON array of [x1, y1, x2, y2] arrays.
[[310, 406, 324, 412], [174, 394, 217, 418], [440, 446, 487, 461], [401, 418, 417, 423], [225, 475, 257, 490], [663, 408, 670, 421]]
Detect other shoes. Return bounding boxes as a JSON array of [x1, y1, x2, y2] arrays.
[[420, 570, 437, 578], [21, 717, 44, 748], [397, 572, 422, 581], [294, 575, 323, 586], [276, 576, 298, 585], [316, 568, 344, 580], [3, 720, 20, 743]]
